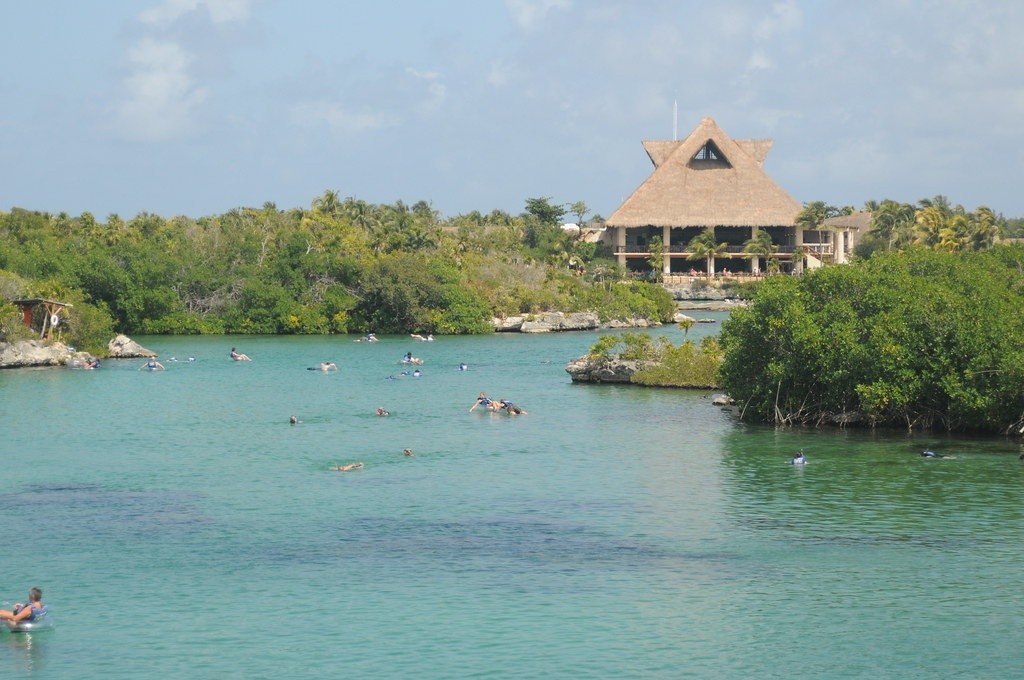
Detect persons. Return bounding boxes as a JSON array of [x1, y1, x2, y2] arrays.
[[320, 361, 336, 370], [376, 406, 389, 416], [401, 352, 422, 366], [84, 357, 100, 369], [411, 332, 433, 341], [470, 392, 526, 416], [138, 356, 165, 371], [0, 587, 44, 626], [231, 347, 252, 360], [689, 267, 803, 277], [353, 333, 381, 342], [788, 449, 807, 465]]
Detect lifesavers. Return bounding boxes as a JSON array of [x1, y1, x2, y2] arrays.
[[6, 620, 54, 633], [50, 315, 58, 325]]
